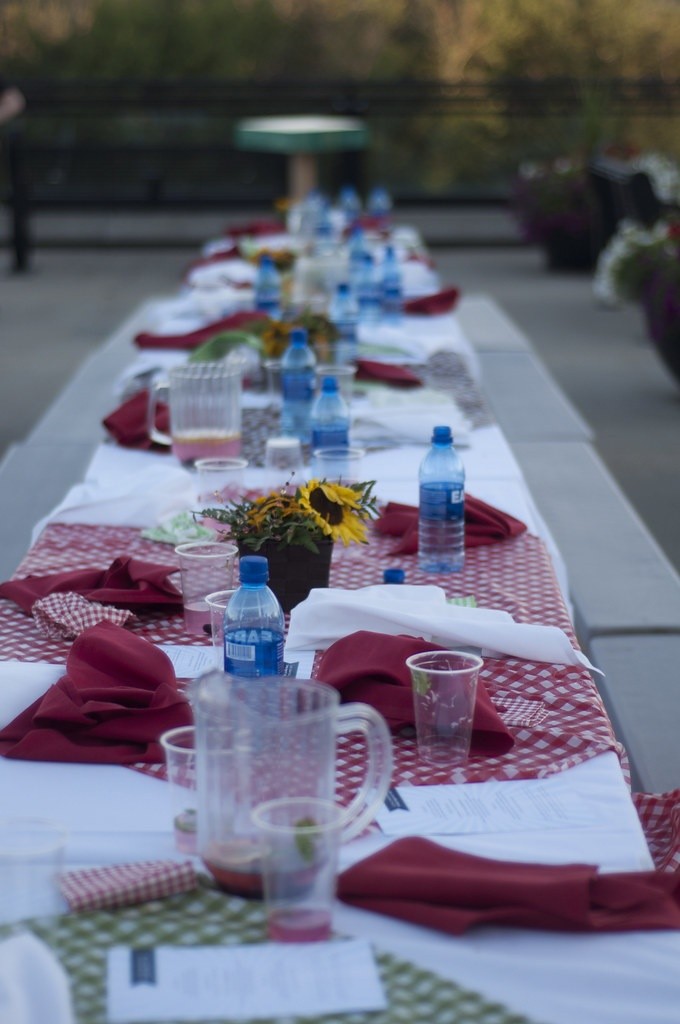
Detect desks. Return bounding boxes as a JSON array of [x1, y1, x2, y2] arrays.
[[0, 220, 680, 1024], [233, 116, 374, 217]]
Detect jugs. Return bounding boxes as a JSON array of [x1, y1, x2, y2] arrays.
[[148, 361, 247, 469], [185, 671, 391, 900]]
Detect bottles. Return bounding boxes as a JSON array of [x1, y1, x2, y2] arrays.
[[255, 257, 283, 321], [281, 328, 315, 445], [310, 378, 348, 477], [297, 188, 402, 361], [419, 425, 465, 573], [223, 556, 285, 680]]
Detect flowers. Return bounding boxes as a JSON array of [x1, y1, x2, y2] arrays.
[[191, 470, 382, 555]]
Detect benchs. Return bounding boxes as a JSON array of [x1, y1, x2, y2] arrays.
[[0, 294, 171, 591], [455, 295, 680, 795]]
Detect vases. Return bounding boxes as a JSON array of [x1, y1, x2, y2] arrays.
[[237, 539, 334, 613]]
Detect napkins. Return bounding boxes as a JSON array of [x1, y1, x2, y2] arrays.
[[186, 245, 241, 265], [336, 837, 680, 935], [135, 311, 272, 352], [30, 465, 191, 546], [0, 618, 195, 763], [284, 585, 605, 677], [354, 360, 422, 388], [342, 407, 471, 450], [398, 289, 461, 315], [101, 390, 172, 454], [315, 630, 515, 759], [340, 216, 389, 237], [0, 555, 184, 618], [222, 223, 279, 239]]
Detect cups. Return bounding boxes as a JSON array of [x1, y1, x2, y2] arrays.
[[312, 446, 364, 486], [406, 650, 482, 763], [260, 437, 307, 499], [206, 588, 236, 662], [160, 724, 239, 854], [175, 542, 238, 636], [316, 364, 354, 408], [266, 360, 284, 415], [253, 796, 353, 946], [195, 457, 249, 531]]
